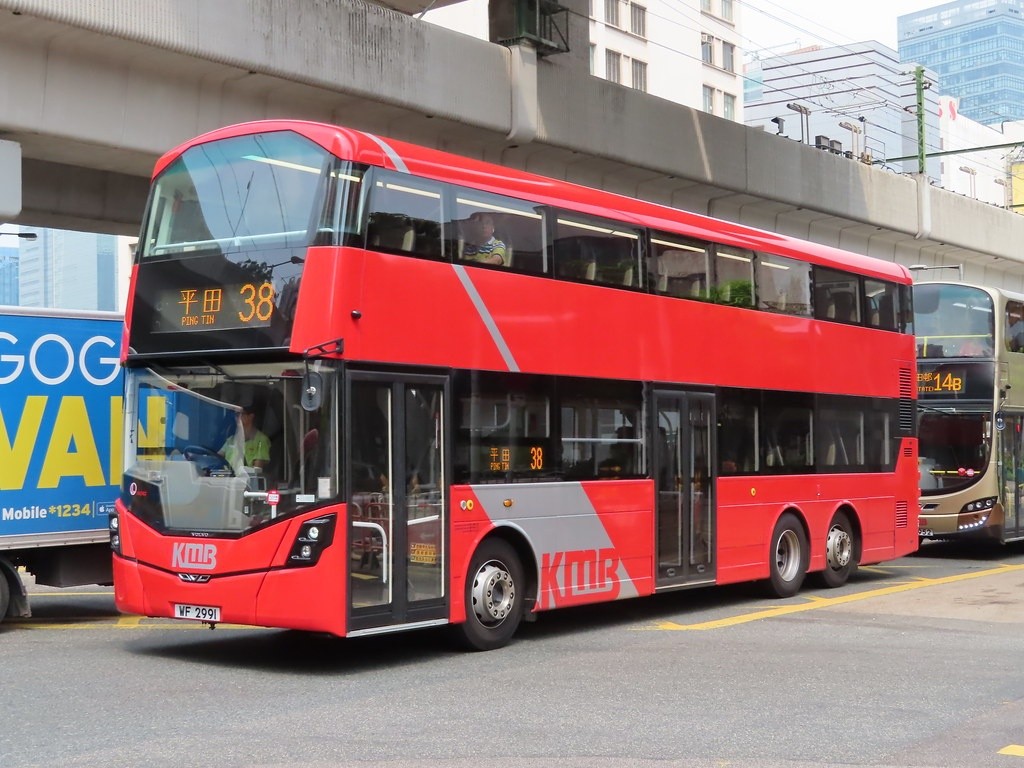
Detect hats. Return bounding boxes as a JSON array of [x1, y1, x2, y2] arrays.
[[234, 400, 253, 413]]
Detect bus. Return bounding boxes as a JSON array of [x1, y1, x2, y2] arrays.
[[858, 282, 1024, 549], [108, 115, 921, 653]]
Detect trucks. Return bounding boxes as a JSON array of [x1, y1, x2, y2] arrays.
[[0, 307, 200, 617]]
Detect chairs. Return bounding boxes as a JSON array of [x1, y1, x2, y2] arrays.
[[742, 426, 884, 472], [371, 213, 882, 328]]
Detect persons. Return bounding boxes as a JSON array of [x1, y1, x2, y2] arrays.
[[183, 404, 271, 469], [463, 215, 507, 265], [1006, 309, 1024, 352]]
